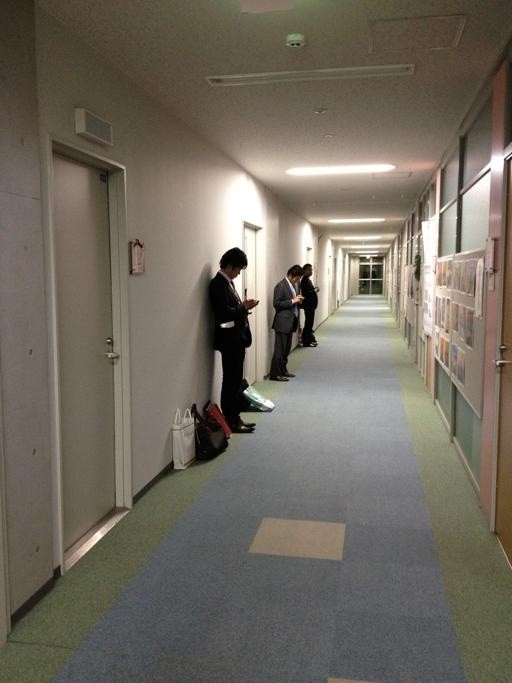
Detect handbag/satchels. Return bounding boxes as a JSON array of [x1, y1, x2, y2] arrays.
[[190, 403, 228, 461], [171, 407, 198, 471], [239, 378, 275, 412], [301, 327, 317, 342], [202, 399, 231, 439]]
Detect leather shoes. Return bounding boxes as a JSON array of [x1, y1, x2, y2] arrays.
[[227, 420, 257, 433], [270, 372, 296, 381], [302, 341, 318, 347]]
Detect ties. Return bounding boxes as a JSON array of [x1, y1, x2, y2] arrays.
[[230, 280, 243, 305]]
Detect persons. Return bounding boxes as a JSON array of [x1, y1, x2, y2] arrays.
[[268, 264, 306, 380], [300, 263, 320, 346], [207, 247, 259, 433]]
